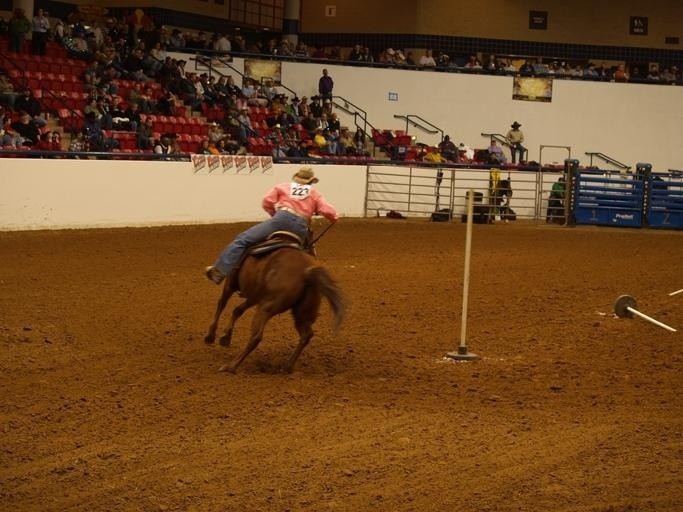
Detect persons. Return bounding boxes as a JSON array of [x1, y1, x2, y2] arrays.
[[205, 166, 338, 284], [380, 47, 682, 85], [546, 177, 566, 223], [492, 180, 509, 223], [1, 2, 373, 165], [387, 120, 525, 165]]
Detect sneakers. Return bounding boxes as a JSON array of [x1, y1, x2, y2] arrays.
[[206, 264, 224, 284]]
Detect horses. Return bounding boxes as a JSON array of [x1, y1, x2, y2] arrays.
[[203, 235, 353, 374]]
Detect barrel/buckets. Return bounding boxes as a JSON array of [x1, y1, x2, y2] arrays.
[[465, 191, 483, 215]]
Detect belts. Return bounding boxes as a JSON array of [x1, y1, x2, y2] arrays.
[[282, 207, 309, 222]]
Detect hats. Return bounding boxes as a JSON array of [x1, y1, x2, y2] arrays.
[[200, 73, 208, 78], [209, 121, 220, 128], [273, 123, 283, 129], [511, 120, 521, 128], [177, 60, 187, 66], [292, 167, 318, 183], [272, 93, 321, 102]]
